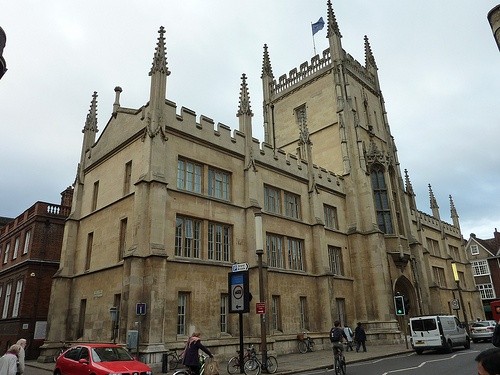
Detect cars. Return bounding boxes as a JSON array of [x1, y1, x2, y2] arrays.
[[54, 344, 152, 375], [471, 320, 497, 342]]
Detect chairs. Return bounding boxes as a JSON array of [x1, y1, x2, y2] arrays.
[[104, 350, 115, 359]]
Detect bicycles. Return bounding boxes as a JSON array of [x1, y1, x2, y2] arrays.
[[172, 355, 213, 375], [333, 341, 349, 375], [297, 331, 316, 353], [226, 343, 278, 375]]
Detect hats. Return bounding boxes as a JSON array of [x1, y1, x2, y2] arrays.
[[357, 322, 362, 326]]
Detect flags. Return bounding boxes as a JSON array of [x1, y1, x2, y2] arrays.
[[312, 17, 325, 35]]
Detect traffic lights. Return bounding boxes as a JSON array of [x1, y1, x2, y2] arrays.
[[394, 296, 405, 315]]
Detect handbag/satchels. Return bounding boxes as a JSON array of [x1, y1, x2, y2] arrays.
[[348, 327, 354, 337]]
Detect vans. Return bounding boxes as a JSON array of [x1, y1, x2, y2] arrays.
[[408, 314, 470, 354]]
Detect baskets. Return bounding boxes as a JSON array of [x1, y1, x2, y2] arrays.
[[297, 335, 304, 341]]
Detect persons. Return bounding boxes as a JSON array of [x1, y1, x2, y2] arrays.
[[330, 320, 348, 373], [15, 338, 27, 375], [182, 329, 214, 375], [0, 344, 22, 375], [342, 324, 354, 352], [474, 347, 500, 375], [492, 321, 500, 347], [355, 322, 367, 352]]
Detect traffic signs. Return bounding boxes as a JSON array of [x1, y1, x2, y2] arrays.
[[254, 302, 265, 315]]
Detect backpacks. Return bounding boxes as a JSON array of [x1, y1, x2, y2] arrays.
[[330, 328, 342, 342]]
